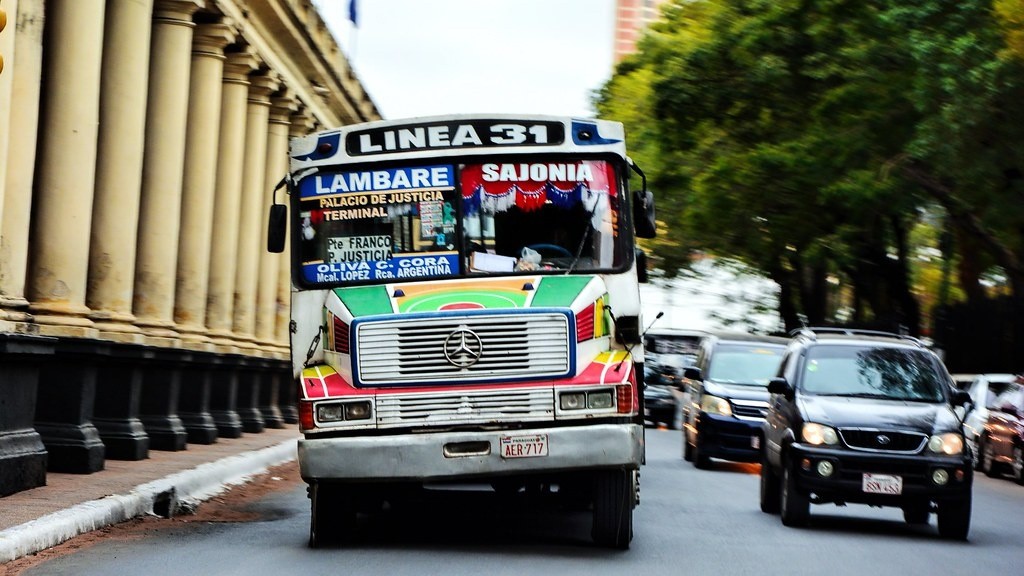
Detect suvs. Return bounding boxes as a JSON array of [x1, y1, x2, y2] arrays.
[[759, 327, 973, 543]]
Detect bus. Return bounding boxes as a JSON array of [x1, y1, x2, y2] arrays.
[[265, 112, 656, 550]]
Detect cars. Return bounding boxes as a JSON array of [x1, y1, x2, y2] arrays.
[[643, 330, 704, 426], [949, 369, 1023, 484]]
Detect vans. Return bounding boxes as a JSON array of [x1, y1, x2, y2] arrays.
[[678, 328, 790, 472]]
